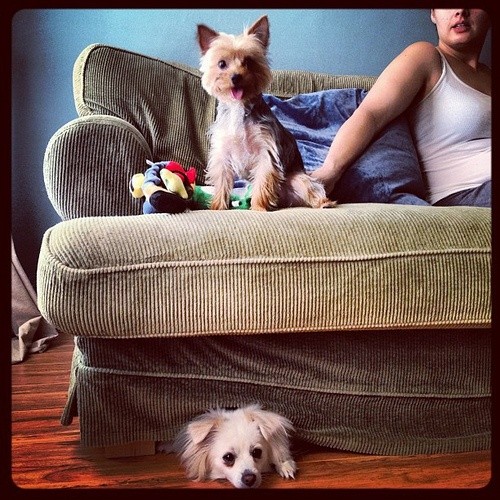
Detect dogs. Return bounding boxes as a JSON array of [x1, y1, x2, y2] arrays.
[[195, 14, 337, 213], [170, 399, 297, 489]]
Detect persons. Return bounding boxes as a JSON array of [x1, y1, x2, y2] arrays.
[[309, 7, 491, 206]]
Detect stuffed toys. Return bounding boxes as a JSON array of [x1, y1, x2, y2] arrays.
[[129, 160, 197, 214]]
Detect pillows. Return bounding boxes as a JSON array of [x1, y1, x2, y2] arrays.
[[265, 88, 433, 208]]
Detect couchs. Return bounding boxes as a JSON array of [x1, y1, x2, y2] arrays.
[[35, 43, 491, 457]]
